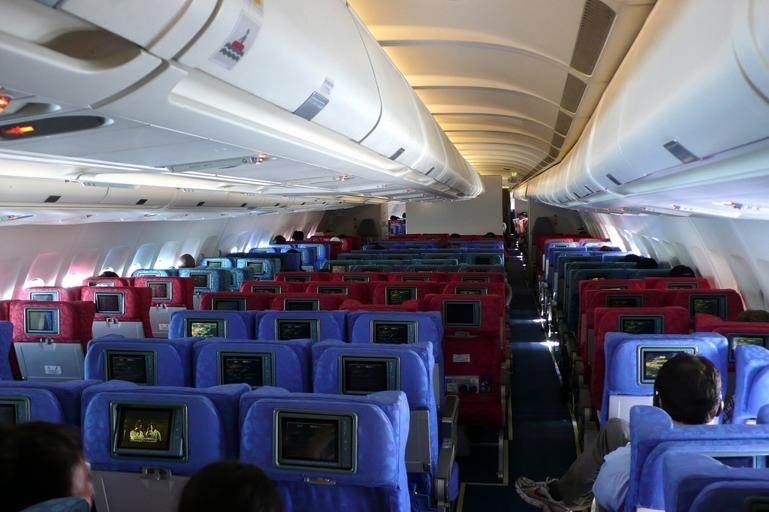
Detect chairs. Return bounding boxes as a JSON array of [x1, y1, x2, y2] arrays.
[[514, 476, 594, 512], [532, 228, 769, 512], [1, 230, 512, 511]]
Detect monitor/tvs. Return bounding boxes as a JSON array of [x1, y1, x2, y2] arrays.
[[0, 395, 31, 427], [110, 401, 188, 462], [250, 284, 282, 294], [400, 275, 430, 281], [268, 257, 283, 275], [342, 275, 369, 282], [454, 286, 488, 297], [442, 299, 482, 328], [284, 297, 320, 311], [26, 308, 59, 334], [338, 354, 401, 396], [384, 286, 417, 305], [461, 276, 490, 282], [273, 409, 358, 473], [190, 272, 211, 289], [102, 349, 157, 387], [216, 350, 275, 391], [228, 272, 237, 288], [329, 263, 347, 273], [728, 332, 769, 363], [284, 275, 312, 282], [212, 297, 247, 312], [370, 319, 418, 342], [636, 345, 698, 385], [207, 261, 221, 269], [183, 318, 228, 339], [316, 286, 349, 295], [95, 292, 124, 315], [29, 291, 60, 301], [247, 261, 264, 275], [274, 318, 320, 342], [146, 281, 173, 301], [597, 284, 727, 335]]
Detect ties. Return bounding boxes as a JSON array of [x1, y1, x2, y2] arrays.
[[273, 409, 358, 473], [636, 345, 698, 385], [102, 349, 157, 387], [597, 284, 727, 335], [0, 395, 31, 427], [110, 401, 188, 462], [338, 354, 401, 396], [216, 350, 275, 391], [728, 332, 769, 363]]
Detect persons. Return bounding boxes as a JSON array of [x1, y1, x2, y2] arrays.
[[291, 230, 305, 242], [329, 237, 343, 241], [178, 254, 196, 268], [665, 264, 696, 278], [511, 352, 724, 511], [174, 458, 290, 512], [511, 209, 529, 265], [388, 213, 407, 225], [128, 418, 145, 441], [599, 246, 613, 251], [143, 420, 161, 442], [632, 256, 659, 268], [98, 271, 119, 278], [272, 235, 286, 244], [622, 254, 640, 262], [1, 418, 98, 511]]
